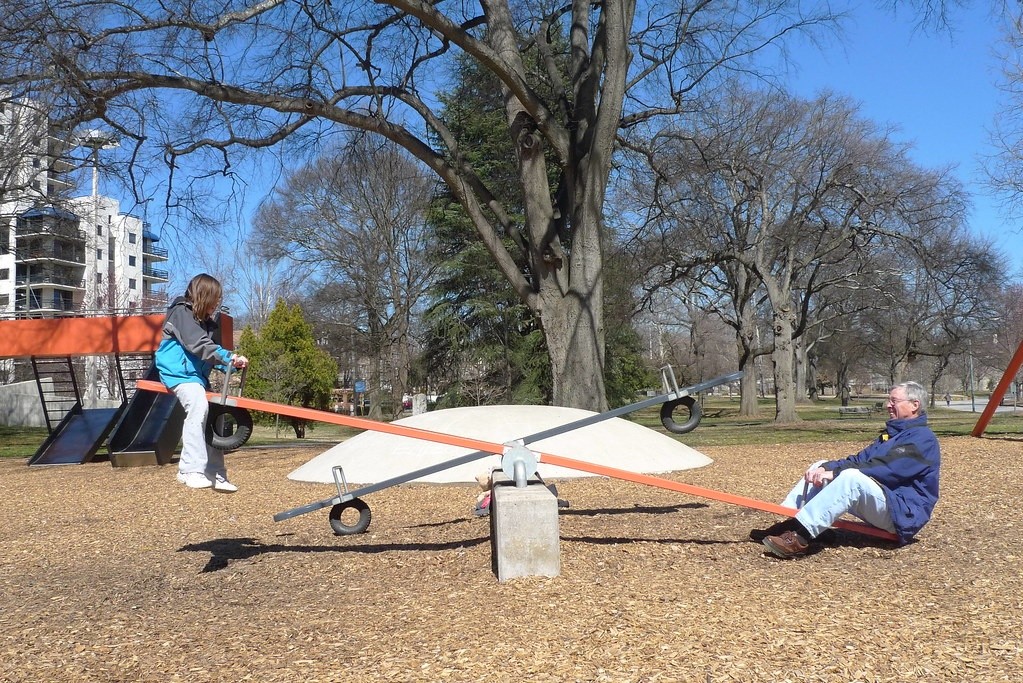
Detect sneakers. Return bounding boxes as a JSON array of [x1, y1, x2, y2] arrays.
[[205, 468, 238, 493], [749, 521, 794, 542], [176, 470, 213, 488], [762, 530, 810, 559]]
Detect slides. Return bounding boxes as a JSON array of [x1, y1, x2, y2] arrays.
[[105, 312, 221, 469], [25, 399, 128, 467]]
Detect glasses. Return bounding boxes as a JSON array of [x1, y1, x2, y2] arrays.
[[216, 296, 223, 302], [884, 396, 913, 406]]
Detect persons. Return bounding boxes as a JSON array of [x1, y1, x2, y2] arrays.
[[402, 393, 412, 410], [944, 392, 951, 407], [154, 273, 248, 494], [749, 381, 939, 558], [988, 390, 1005, 406]]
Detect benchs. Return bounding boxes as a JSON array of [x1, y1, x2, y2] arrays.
[[839, 407, 872, 419], [872, 402, 884, 413]]
